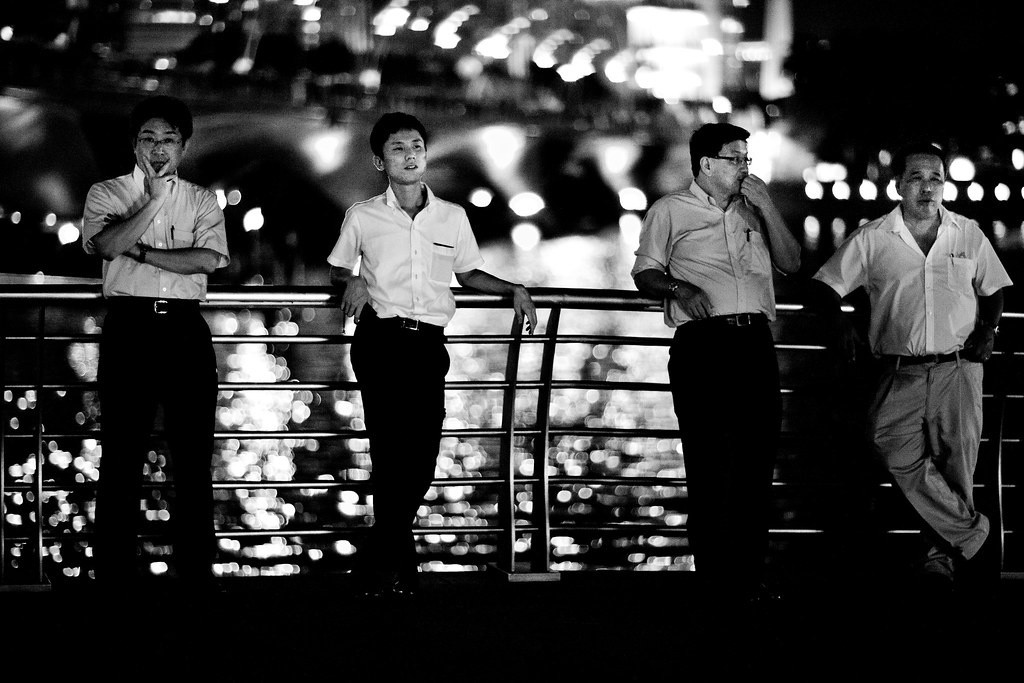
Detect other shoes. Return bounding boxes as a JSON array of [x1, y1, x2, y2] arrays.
[[331, 550, 377, 593], [747, 584, 783, 602], [385, 558, 424, 600], [175, 570, 224, 593], [913, 530, 956, 584], [949, 532, 994, 596]]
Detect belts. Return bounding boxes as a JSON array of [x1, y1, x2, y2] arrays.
[[877, 355, 982, 370], [681, 313, 769, 330], [107, 296, 200, 320], [376, 316, 445, 336]]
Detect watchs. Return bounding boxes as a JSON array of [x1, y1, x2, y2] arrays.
[[668, 280, 685, 290]]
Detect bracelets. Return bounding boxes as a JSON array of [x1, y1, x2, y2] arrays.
[[139, 244, 152, 263]]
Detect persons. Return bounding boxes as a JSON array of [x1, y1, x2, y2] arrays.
[[326, 110, 537, 564], [80, 102, 234, 558], [635, 122, 803, 571], [809, 150, 1014, 579]]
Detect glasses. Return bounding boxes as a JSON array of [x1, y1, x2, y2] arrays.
[[137, 136, 184, 152], [706, 153, 748, 169]]
